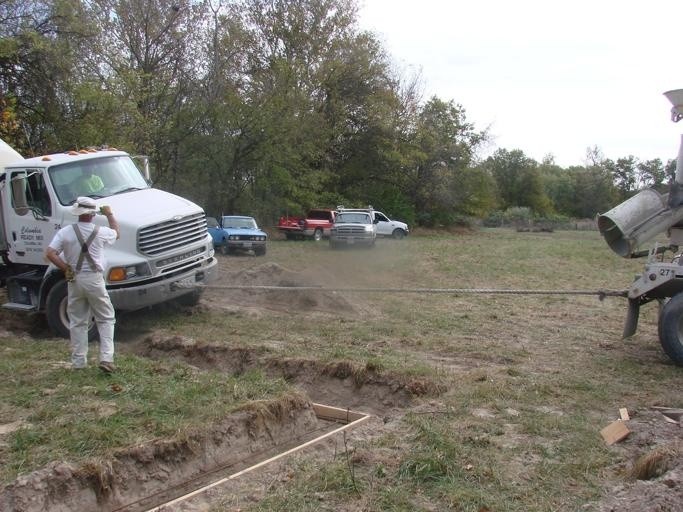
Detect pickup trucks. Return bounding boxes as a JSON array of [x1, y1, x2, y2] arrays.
[[271, 209, 343, 241]]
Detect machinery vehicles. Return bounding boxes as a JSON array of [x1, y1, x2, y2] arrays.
[[0, 137, 220, 341], [595, 88, 681, 370]]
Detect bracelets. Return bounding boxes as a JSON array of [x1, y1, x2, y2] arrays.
[[106, 212, 114, 218]]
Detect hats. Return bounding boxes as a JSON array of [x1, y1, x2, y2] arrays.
[[71, 196, 99, 215]]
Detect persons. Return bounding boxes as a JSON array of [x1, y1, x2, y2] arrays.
[[46, 197, 120, 373], [69, 165, 104, 200]]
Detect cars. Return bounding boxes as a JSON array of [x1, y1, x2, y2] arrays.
[[203, 214, 267, 256]]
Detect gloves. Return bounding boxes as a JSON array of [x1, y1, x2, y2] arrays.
[[64, 267, 75, 281], [99, 205, 113, 217]]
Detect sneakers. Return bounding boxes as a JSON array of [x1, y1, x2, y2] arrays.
[[99, 361, 116, 371]]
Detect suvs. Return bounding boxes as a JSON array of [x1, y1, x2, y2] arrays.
[[328, 212, 378, 250], [334, 204, 409, 240]]
